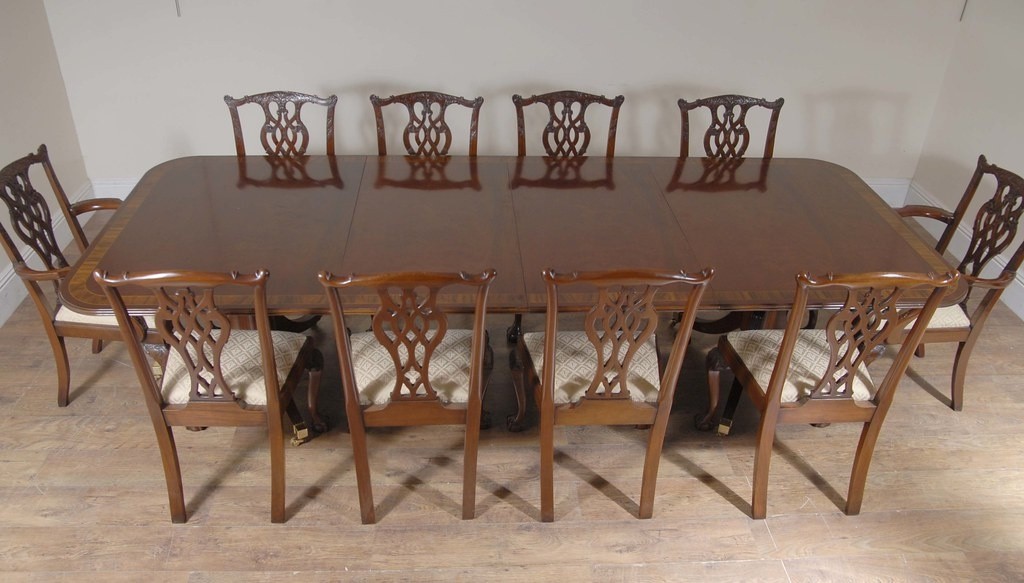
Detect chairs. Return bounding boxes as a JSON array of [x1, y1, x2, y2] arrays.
[[694, 269, 960, 520], [508, 266, 715, 522], [512, 90, 625, 157], [223, 91, 338, 157], [370, 92, 483, 155], [678, 95, 784, 158], [318, 267, 497, 526], [806, 155, 1024, 412], [90, 268, 328, 523], [0, 142, 169, 409]]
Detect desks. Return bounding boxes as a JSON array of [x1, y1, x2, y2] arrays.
[[56, 155, 968, 332]]
